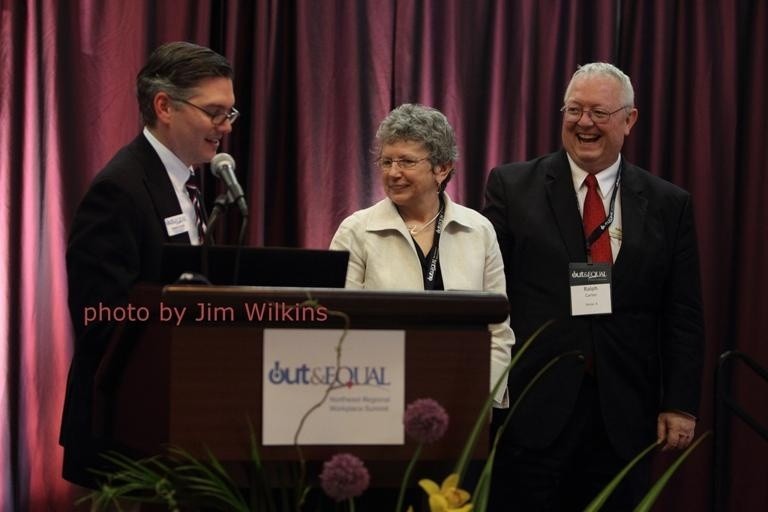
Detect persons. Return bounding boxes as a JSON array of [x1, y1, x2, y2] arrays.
[[324, 100, 519, 474], [55, 42, 246, 499], [477, 58, 712, 510]]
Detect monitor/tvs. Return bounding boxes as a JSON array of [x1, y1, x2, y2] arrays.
[[166, 244, 349, 287]]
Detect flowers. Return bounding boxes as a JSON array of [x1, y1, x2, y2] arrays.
[[71, 291, 711, 512]]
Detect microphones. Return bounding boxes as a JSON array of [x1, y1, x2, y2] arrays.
[[210, 153, 249, 217]]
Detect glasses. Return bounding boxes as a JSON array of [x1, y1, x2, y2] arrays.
[[373, 155, 430, 170], [171, 97, 240, 126], [560, 105, 630, 123]]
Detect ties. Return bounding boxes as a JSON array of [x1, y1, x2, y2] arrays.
[[186, 175, 208, 246], [582, 175, 613, 266]]
